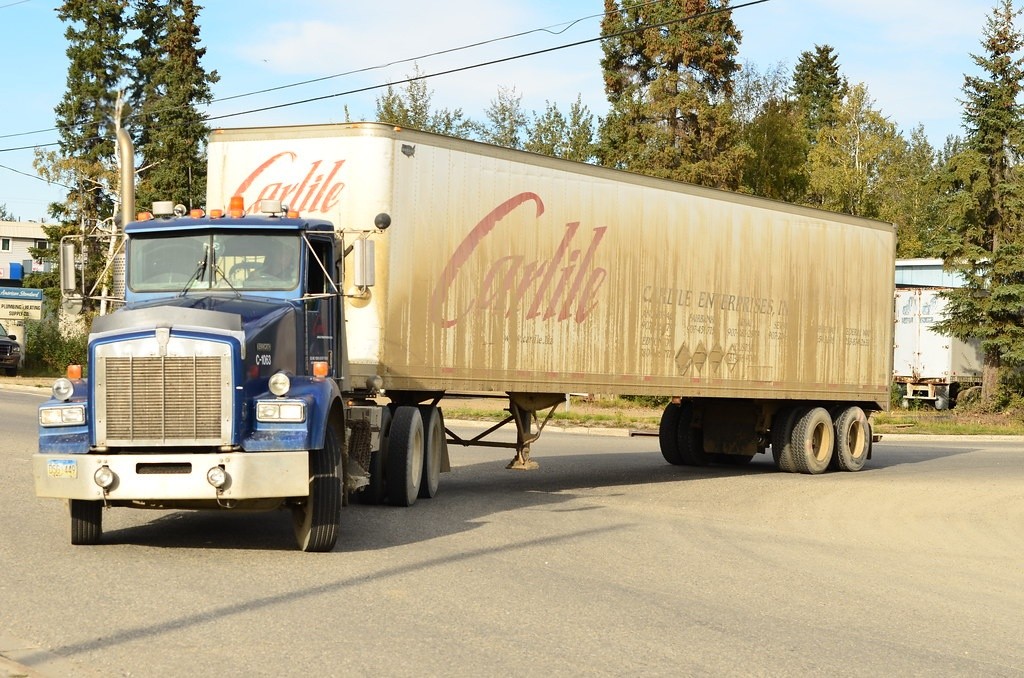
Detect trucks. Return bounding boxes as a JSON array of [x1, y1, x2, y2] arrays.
[[895, 259, 1008, 411]]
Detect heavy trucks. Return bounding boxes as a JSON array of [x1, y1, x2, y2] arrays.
[[31, 121, 900, 555]]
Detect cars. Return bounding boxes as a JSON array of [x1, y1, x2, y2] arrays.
[[0, 323, 21, 377]]
